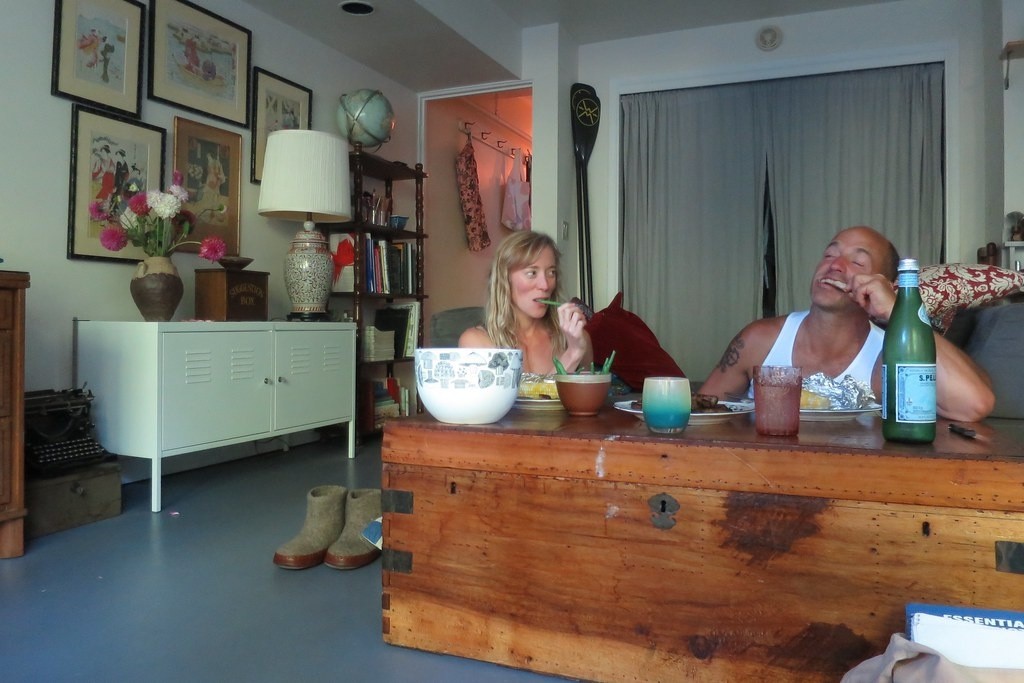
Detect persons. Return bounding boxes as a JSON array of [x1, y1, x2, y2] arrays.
[[701, 225, 995, 422], [458, 230, 594, 372]]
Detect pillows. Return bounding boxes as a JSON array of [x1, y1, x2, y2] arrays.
[[584, 291, 687, 393], [891, 262, 1024, 337]]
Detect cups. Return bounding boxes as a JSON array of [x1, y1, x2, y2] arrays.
[[368, 208, 390, 226], [642, 377, 692, 434], [753, 366, 803, 436]]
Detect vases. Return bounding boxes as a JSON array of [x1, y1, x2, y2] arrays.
[[128, 256, 184, 321]]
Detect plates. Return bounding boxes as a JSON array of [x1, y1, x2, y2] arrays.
[[800, 405, 882, 422], [515, 399, 561, 402], [615, 400, 755, 425]]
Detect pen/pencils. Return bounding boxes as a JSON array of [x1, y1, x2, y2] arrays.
[[362, 189, 385, 226]]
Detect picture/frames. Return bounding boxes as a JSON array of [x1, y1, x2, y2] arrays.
[[249, 65, 314, 186], [50, 0, 147, 120], [146, 0, 253, 131], [67, 102, 167, 266], [169, 116, 243, 258]]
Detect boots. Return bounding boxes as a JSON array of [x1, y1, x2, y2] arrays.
[[325, 488, 382, 570], [273, 485, 348, 570]]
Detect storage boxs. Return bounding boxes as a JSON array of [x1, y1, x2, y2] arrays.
[[24, 460, 123, 541]]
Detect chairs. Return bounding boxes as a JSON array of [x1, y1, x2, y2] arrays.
[[428, 305, 489, 348], [886, 259, 1024, 416]]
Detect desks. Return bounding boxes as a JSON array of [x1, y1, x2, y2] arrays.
[[381, 391, 1024, 683], [0, 270, 32, 557]]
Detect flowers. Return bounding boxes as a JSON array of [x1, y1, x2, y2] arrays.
[[87, 171, 229, 265]]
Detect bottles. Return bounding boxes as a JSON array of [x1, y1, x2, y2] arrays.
[[882, 259, 937, 444]]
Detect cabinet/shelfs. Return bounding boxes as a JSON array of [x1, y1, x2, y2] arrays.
[[313, 141, 429, 449], [71, 317, 357, 513]]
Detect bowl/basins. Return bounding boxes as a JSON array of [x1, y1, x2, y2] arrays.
[[414, 348, 524, 425], [218, 256, 254, 270], [390, 217, 409, 230], [554, 371, 612, 415]]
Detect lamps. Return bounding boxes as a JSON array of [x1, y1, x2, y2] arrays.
[[257, 128, 352, 320]]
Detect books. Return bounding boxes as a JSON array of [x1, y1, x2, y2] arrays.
[[329, 232, 417, 294], [375, 301, 420, 357], [373, 377, 410, 425]]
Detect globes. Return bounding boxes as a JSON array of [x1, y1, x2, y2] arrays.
[[338, 89, 395, 153]]
